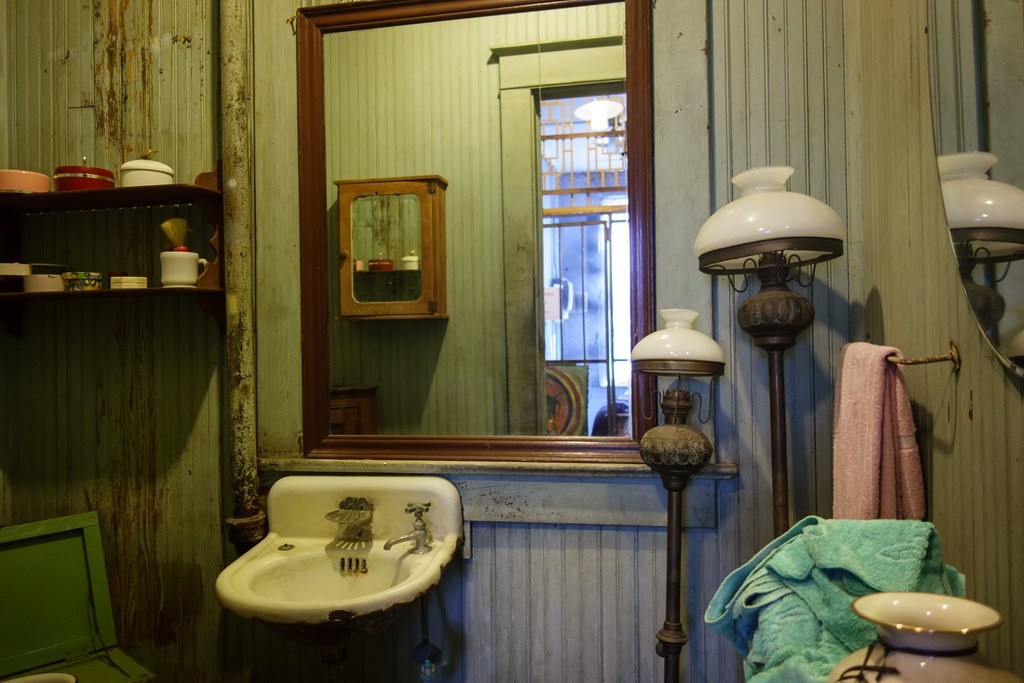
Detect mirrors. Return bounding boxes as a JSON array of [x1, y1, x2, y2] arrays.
[[350, 194, 422, 302], [295, 0, 661, 466], [925, 0, 1024, 388]]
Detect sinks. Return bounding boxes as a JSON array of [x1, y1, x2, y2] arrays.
[[214, 531, 456, 647]]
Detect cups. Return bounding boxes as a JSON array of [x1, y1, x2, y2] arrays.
[[160, 252, 208, 287]]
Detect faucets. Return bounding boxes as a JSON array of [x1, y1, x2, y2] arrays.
[[383, 501, 433, 554]]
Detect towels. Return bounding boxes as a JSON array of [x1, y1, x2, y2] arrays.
[[702, 514, 970, 683], [831, 339, 929, 521]]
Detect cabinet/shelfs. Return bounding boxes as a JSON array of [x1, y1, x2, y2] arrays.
[[0, 183, 224, 298]]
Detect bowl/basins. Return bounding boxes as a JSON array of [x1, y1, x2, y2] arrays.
[[0, 169, 52, 193], [54, 158, 115, 192], [368, 254, 394, 273], [401, 250, 419, 270], [119, 150, 175, 187]]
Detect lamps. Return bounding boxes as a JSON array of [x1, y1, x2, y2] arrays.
[[939, 151, 1024, 331], [630, 307, 727, 683], [695, 164, 846, 542]]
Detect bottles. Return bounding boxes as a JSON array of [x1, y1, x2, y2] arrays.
[[829, 591, 1024, 682]]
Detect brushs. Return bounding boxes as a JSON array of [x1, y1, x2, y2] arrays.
[[161, 218, 189, 252]]
